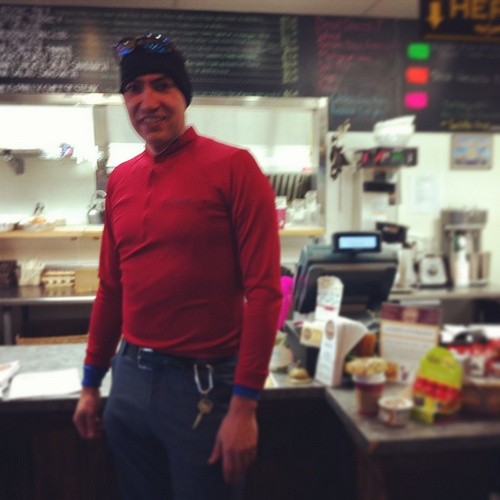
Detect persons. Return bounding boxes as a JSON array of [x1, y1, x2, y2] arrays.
[[72, 37, 282, 500]]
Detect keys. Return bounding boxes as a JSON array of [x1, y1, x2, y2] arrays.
[[191, 397, 213, 432]]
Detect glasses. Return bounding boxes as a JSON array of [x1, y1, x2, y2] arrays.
[[111, 30, 175, 64]]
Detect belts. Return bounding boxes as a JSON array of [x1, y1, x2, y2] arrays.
[[124, 345, 237, 372]]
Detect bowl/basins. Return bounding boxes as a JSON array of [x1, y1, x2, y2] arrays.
[[375, 395, 415, 428]]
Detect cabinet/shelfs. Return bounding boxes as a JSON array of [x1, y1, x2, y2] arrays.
[[0, 102, 323, 237]]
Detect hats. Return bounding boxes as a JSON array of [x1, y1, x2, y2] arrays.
[[117, 38, 192, 108]]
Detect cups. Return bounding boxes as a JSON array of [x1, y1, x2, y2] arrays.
[[277, 209, 285, 229], [351, 373, 387, 418]]
